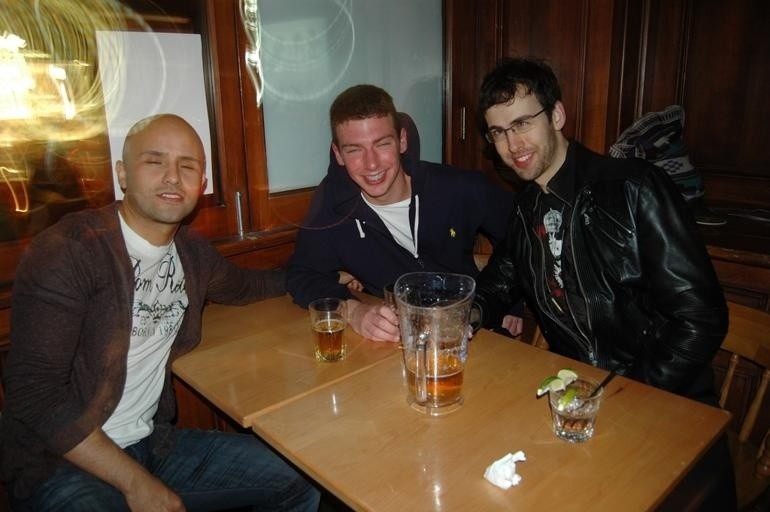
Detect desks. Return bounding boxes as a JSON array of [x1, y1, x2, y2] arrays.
[[170, 293, 735, 512], [169, 293, 734, 511]]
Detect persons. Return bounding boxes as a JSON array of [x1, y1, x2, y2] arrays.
[[411, 56, 728, 479], [0, 115, 365, 512], [287, 84, 526, 343]]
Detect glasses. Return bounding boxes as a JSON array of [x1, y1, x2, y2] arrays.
[[485, 109, 544, 143]]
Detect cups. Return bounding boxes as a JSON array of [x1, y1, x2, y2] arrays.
[[382, 282, 400, 327], [305, 295, 351, 366], [392, 271, 479, 418], [547, 373, 605, 446]]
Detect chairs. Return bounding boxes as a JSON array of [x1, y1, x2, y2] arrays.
[[531, 246, 770, 511]]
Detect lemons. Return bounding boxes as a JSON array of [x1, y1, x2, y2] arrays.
[[557, 368, 578, 386], [536, 376, 566, 396], [560, 390, 578, 406]]
